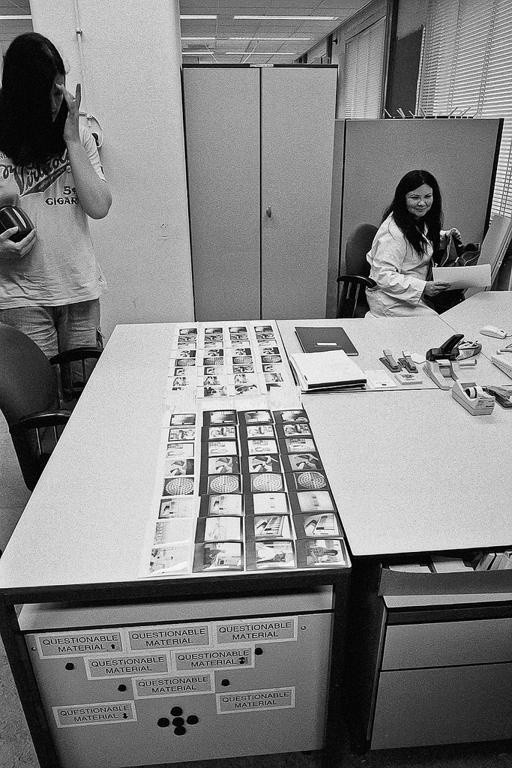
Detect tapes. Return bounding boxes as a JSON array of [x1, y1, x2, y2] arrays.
[[464, 385, 483, 399]]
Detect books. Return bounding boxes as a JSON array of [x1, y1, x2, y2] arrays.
[[288, 326, 367, 394]]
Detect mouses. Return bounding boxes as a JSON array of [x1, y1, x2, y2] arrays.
[[479, 326, 508, 339]]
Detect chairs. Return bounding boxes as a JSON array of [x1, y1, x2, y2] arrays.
[[335, 222, 378, 318]]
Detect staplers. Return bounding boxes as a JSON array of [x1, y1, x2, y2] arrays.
[[379, 349, 418, 373], [481, 385, 512, 408]]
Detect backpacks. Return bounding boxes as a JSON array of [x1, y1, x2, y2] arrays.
[[422, 233, 482, 315]]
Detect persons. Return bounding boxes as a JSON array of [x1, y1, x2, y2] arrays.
[[0, 30, 112, 494], [364, 169, 462, 319]]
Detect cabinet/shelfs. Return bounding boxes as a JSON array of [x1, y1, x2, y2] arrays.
[[341, 116, 504, 276], [181, 63, 340, 321]]
